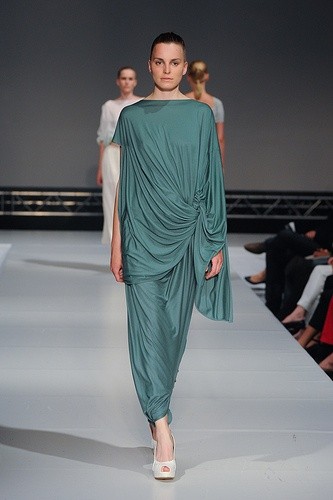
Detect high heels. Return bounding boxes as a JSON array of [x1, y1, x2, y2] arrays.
[[280, 316, 306, 336]]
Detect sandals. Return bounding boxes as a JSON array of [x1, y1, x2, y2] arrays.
[[151, 428, 177, 479]]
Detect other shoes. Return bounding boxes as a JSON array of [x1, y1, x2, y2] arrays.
[[244, 273, 267, 285], [243, 242, 266, 254]]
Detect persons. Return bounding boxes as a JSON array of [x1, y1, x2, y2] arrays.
[[109, 33, 234, 479], [244, 213, 333, 381], [96, 65, 142, 244], [182, 59, 225, 170]]
